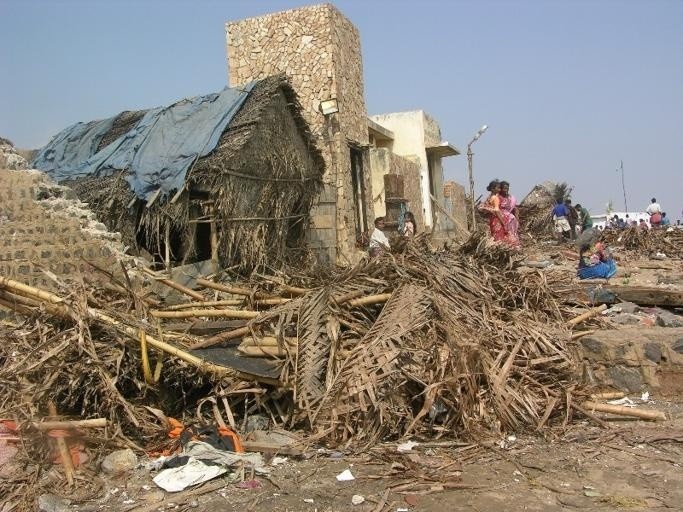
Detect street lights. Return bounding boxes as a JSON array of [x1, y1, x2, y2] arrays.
[[466, 124, 488, 232]]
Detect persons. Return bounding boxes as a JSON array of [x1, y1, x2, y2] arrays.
[[573, 203, 592, 232], [660, 212, 669, 226], [367, 216, 391, 256], [484, 178, 507, 243], [645, 197, 662, 228], [497, 180, 520, 246], [594, 213, 648, 235], [564, 198, 578, 238], [401, 211, 417, 238], [574, 228, 617, 280], [550, 197, 572, 244]]
